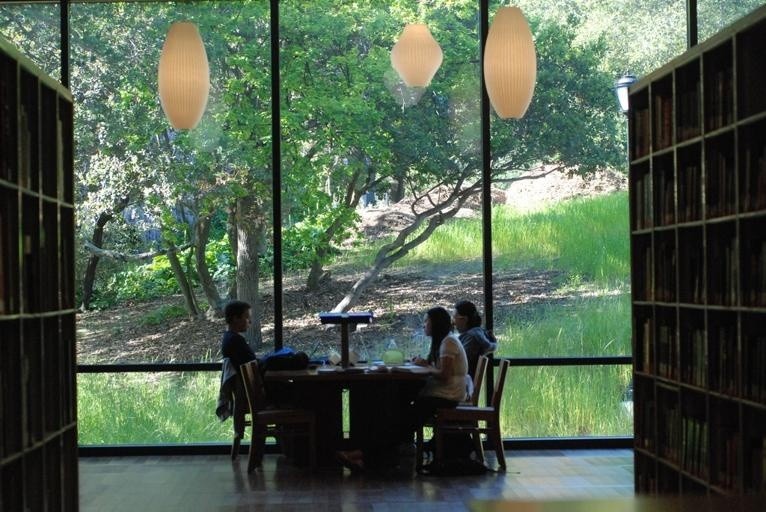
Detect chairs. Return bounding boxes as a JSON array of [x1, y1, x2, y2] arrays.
[[411, 358, 508, 482], [416, 356, 489, 467], [240, 360, 316, 473], [224, 355, 288, 467]]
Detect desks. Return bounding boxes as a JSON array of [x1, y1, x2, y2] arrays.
[[263, 358, 435, 474]]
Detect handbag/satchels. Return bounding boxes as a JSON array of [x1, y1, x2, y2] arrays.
[[260, 348, 308, 370]]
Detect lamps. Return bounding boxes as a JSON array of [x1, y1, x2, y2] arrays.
[[391, 0, 443, 88], [158, 1, 209, 131], [484, 0, 537, 119]]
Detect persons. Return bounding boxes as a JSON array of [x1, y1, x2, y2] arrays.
[[221, 299, 342, 469], [455, 301, 494, 375], [333, 308, 469, 471]]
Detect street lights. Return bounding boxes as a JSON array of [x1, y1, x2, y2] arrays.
[[611, 67, 637, 164]]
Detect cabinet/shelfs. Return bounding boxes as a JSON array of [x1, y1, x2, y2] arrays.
[[628, 4, 766, 497], [0, 36, 79, 512]]
